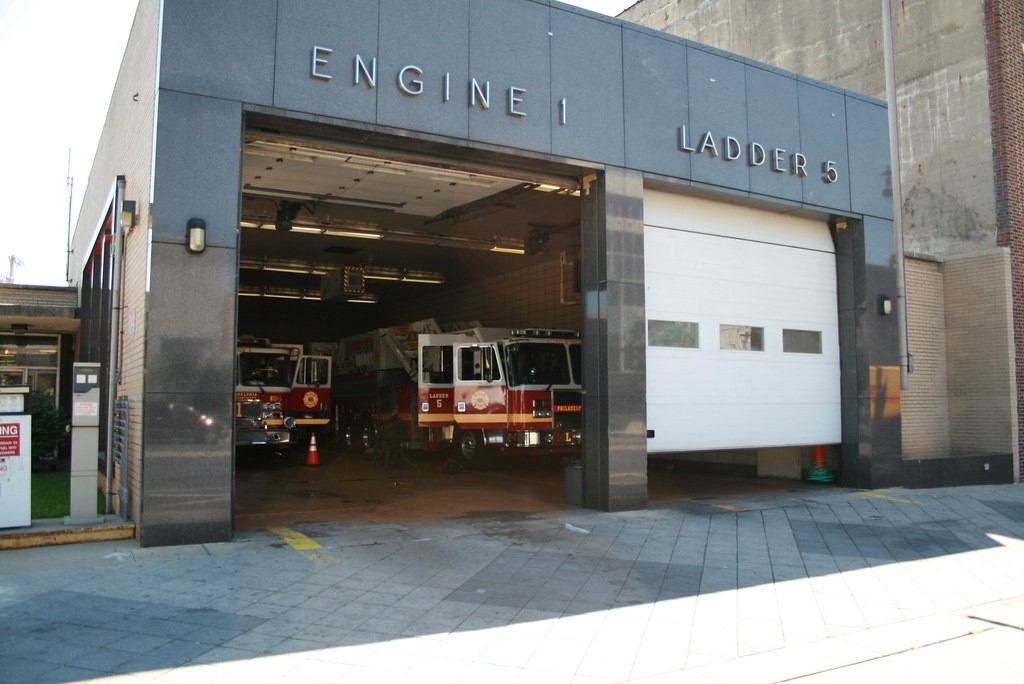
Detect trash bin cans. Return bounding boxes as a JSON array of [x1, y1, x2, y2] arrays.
[[0, 388, 32, 530], [564, 465, 584, 506]]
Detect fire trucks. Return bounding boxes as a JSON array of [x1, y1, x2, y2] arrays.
[[308, 318, 584, 473], [233, 335, 332, 466]]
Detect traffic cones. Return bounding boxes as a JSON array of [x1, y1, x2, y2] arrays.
[[306, 431, 323, 468]]
